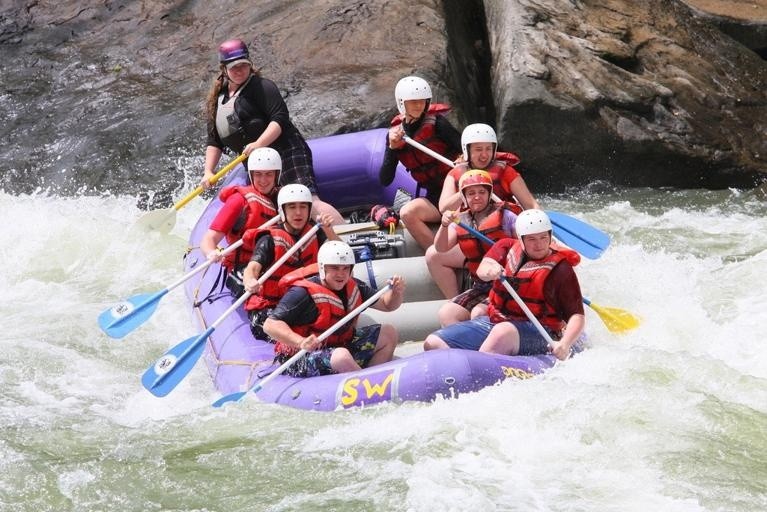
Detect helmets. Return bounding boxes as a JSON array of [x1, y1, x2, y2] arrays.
[[459, 169, 492, 208], [277, 183, 313, 222], [219, 38, 253, 68], [460, 123, 498, 162], [318, 240, 355, 284], [247, 147, 282, 186], [515, 209, 552, 251], [394, 76, 433, 116]]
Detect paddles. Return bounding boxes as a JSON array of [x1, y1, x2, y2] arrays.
[[212, 280, 395, 407], [449, 217, 641, 334], [97, 212, 281, 336], [132, 154, 245, 233], [402, 136, 610, 259], [142, 219, 323, 397]]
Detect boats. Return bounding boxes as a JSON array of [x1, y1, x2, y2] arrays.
[[178, 123, 594, 410]]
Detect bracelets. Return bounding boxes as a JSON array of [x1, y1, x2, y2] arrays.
[[441, 223, 445, 228]]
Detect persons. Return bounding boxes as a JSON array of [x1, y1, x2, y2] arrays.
[[432, 168, 519, 328], [425, 123, 542, 300], [378, 74, 462, 252], [200, 38, 318, 196], [242, 183, 343, 346], [199, 146, 283, 301], [263, 240, 407, 380], [423, 209, 585, 362]]
